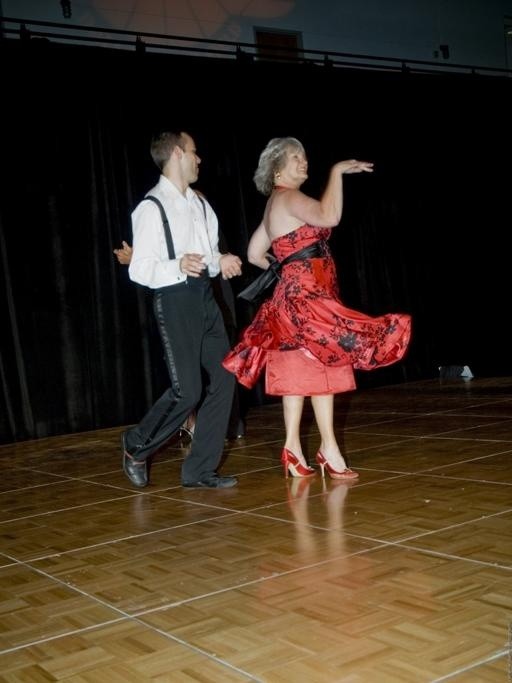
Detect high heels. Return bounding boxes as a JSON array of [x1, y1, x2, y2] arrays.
[[178, 413, 196, 439], [285, 475, 319, 508], [280, 448, 316, 480], [316, 449, 359, 480]]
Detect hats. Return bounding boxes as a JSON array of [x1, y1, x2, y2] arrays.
[[321, 478, 359, 506]]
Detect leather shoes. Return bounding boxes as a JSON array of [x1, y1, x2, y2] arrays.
[[120, 431, 152, 488], [181, 476, 237, 488]]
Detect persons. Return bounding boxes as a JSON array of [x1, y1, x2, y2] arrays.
[[220, 137, 413, 483], [113, 242, 197, 444], [211, 272, 246, 439], [119, 128, 243, 489]]
